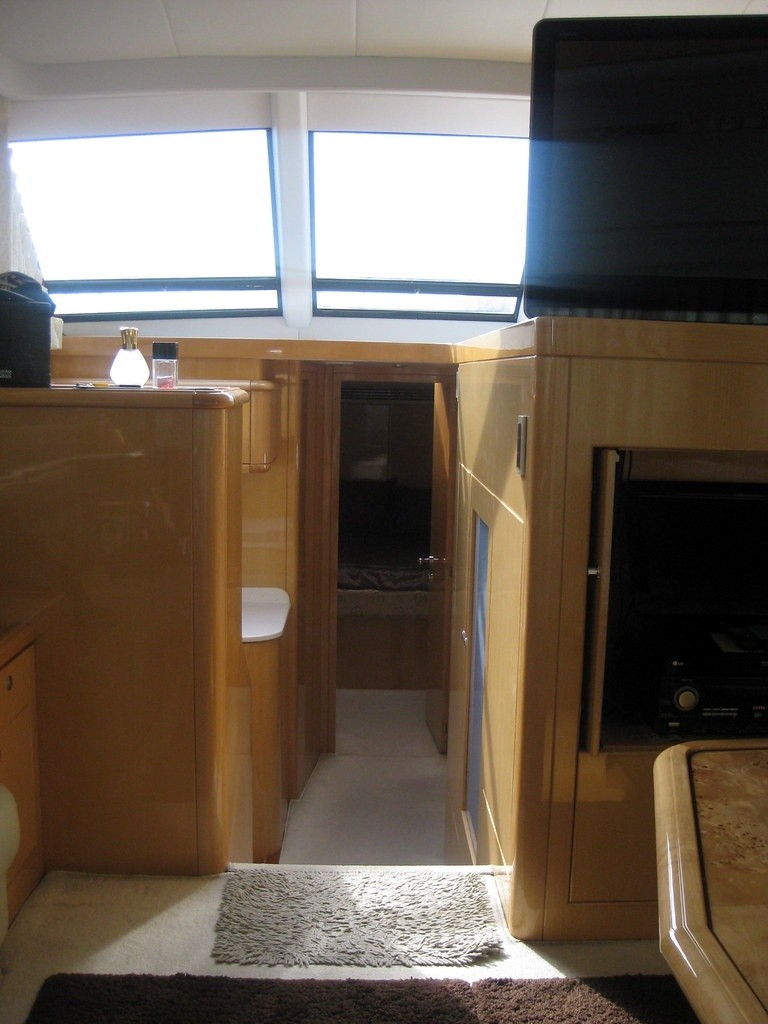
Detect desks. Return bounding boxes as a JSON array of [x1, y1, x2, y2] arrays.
[[651, 738, 768, 1024]]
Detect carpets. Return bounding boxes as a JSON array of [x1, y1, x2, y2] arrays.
[[208, 865, 504, 968], [25, 971, 702, 1024]]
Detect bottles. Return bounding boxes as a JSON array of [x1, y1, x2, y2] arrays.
[[153, 342, 178, 390], [110, 325, 151, 387]]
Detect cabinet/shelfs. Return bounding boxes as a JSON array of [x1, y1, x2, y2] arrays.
[[0, 621, 49, 931]]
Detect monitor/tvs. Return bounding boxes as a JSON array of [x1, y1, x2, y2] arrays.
[[521, 12, 768, 326]]
